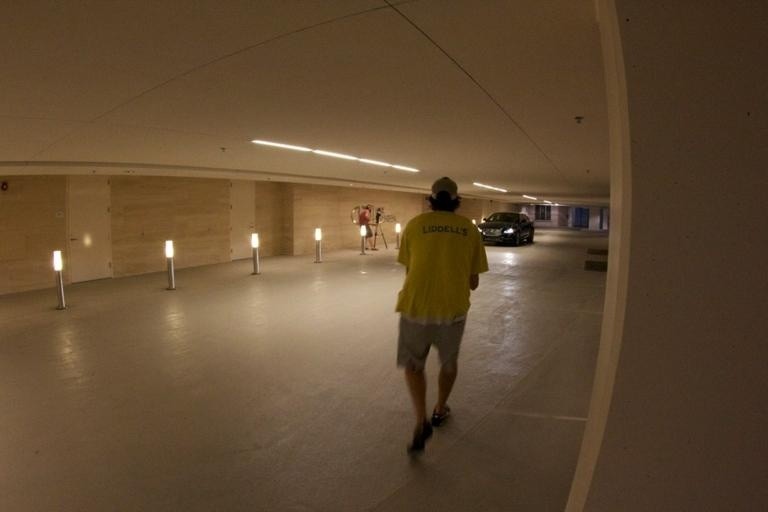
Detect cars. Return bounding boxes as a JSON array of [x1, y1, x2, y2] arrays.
[[476, 210, 535, 246]]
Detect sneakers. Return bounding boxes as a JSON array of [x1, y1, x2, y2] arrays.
[[406, 423, 432, 457], [431, 404, 452, 426]]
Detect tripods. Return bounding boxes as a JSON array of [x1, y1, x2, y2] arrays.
[[373, 215, 388, 249]]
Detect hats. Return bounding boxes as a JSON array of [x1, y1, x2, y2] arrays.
[[423, 176, 462, 201]]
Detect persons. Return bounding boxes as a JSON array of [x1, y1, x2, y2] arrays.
[[359, 206, 374, 249], [394, 175, 490, 456]]
